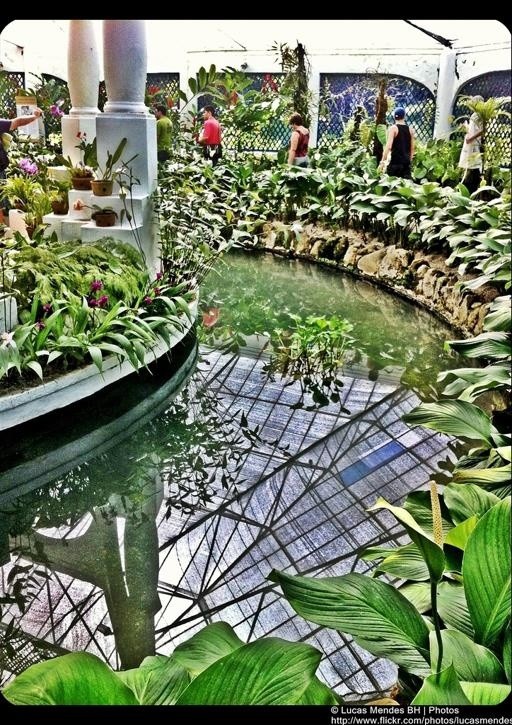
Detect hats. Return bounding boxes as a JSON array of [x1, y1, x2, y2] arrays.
[[393, 107, 406, 120]]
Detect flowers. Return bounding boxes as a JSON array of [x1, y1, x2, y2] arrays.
[[73, 200, 85, 210], [50, 200, 64, 209]]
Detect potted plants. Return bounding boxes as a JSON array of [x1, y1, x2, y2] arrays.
[[87, 138, 140, 196], [55, 154, 95, 190], [88, 204, 119, 226]]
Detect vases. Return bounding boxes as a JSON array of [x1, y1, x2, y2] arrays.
[[72, 208, 91, 220], [54, 208, 68, 214]]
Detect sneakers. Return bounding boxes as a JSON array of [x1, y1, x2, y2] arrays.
[[0, 223, 7, 230]]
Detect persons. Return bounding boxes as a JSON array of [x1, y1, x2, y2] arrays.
[[153, 106, 173, 163], [454, 108, 486, 200], [0, 106, 45, 218], [197, 105, 223, 161], [376, 107, 416, 180], [288, 112, 310, 166]]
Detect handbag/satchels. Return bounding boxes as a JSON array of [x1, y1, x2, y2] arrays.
[[202, 143, 223, 159]]
